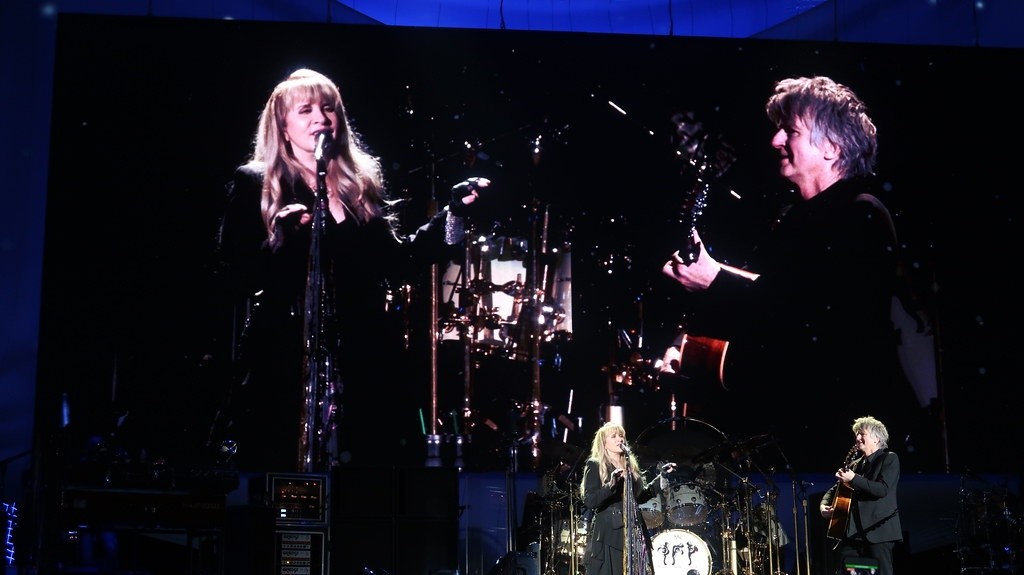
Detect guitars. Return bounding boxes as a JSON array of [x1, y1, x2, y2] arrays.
[[677, 127, 714, 261], [827, 442, 859, 543]]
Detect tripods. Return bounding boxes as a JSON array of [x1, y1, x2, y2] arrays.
[[444, 202, 592, 473]]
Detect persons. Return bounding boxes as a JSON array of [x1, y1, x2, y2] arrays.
[[213, 66, 495, 473], [579, 421, 677, 575], [660, 74, 906, 470], [819, 417, 904, 575]]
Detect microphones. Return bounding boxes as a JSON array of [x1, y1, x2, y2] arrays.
[[620, 443, 629, 455], [315, 131, 331, 160]]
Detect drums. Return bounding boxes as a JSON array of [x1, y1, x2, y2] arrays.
[[650, 528, 714, 575], [665, 480, 709, 527], [640, 481, 663, 529], [431, 233, 550, 360]]
